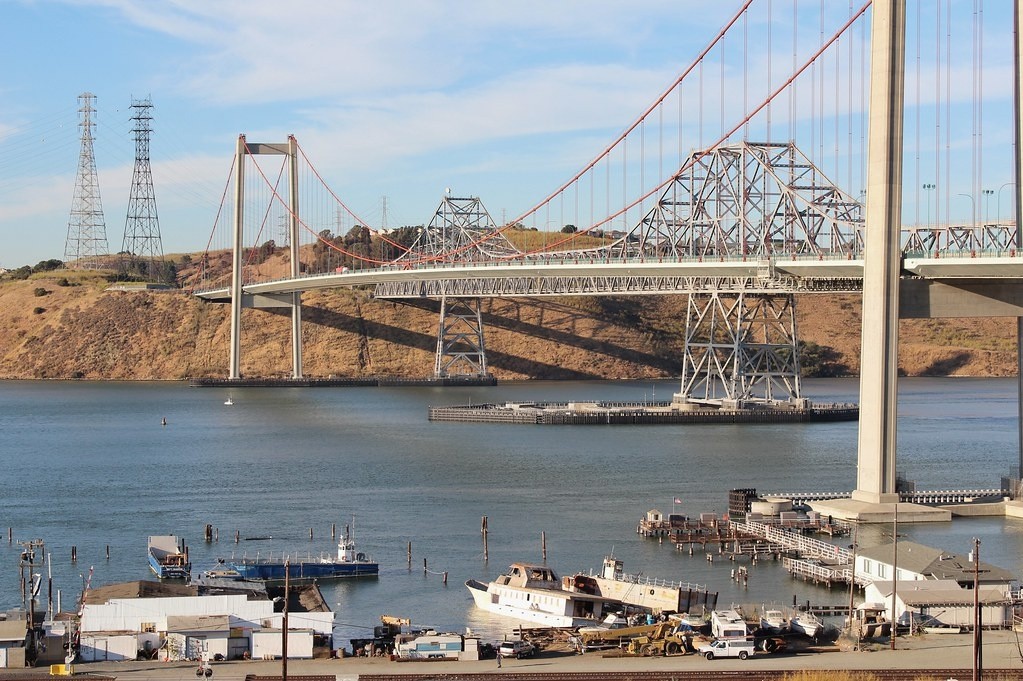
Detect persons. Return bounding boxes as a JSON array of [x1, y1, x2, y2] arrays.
[[496, 650, 501, 668]]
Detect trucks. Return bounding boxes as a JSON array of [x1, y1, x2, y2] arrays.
[[697, 641, 756, 660]]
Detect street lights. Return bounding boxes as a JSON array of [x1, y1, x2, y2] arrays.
[[272, 597, 288, 681]]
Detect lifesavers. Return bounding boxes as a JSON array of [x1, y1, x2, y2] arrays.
[[649, 589, 654, 595]]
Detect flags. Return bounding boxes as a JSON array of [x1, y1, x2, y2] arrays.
[[674, 498, 681, 504]]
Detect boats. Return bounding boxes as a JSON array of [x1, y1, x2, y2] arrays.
[[148, 535, 192, 579], [198, 513, 379, 581], [463, 562, 625, 630]]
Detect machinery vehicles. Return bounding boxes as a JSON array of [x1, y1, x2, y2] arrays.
[[581, 620, 689, 656]]
